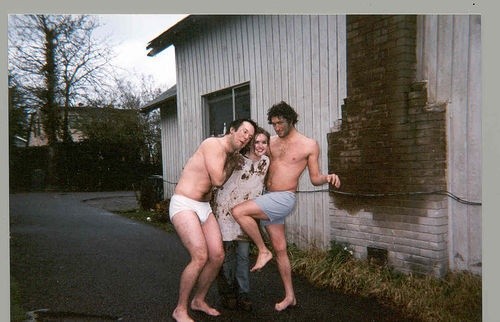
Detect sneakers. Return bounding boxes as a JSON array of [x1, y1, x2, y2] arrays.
[[223, 292, 253, 311]]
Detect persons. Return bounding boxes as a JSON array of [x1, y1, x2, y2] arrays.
[[231, 101, 340, 312], [169, 120, 256, 322], [213, 127, 270, 312]]
